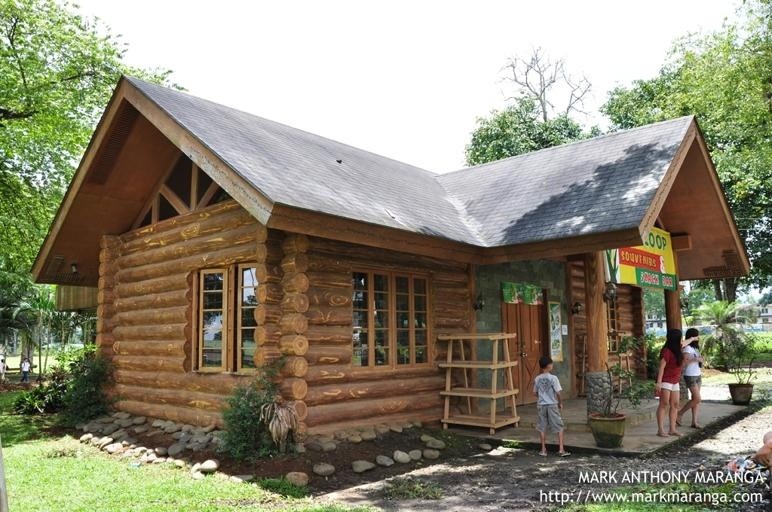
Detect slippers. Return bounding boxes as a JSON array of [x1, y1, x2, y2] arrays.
[[657, 418, 702, 438]]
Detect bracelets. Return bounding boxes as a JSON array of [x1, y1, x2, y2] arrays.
[[656, 382, 661, 384]]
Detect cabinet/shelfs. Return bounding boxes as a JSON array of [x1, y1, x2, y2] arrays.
[[437, 331, 520, 436], [576, 330, 632, 394]]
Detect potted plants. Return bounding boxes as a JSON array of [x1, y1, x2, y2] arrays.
[[588, 362, 626, 448], [717, 332, 772, 406]]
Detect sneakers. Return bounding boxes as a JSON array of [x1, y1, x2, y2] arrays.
[[536, 451, 547, 457], [558, 451, 571, 457]]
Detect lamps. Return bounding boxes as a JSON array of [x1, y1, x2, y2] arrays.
[[571, 302, 582, 315], [602, 281, 618, 302], [472, 291, 485, 312]]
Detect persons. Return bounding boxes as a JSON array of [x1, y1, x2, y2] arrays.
[[20, 358, 32, 382], [755, 431, 772, 491], [675, 328, 704, 430], [654, 328, 699, 438], [0, 359, 6, 381], [531, 357, 572, 457]]
